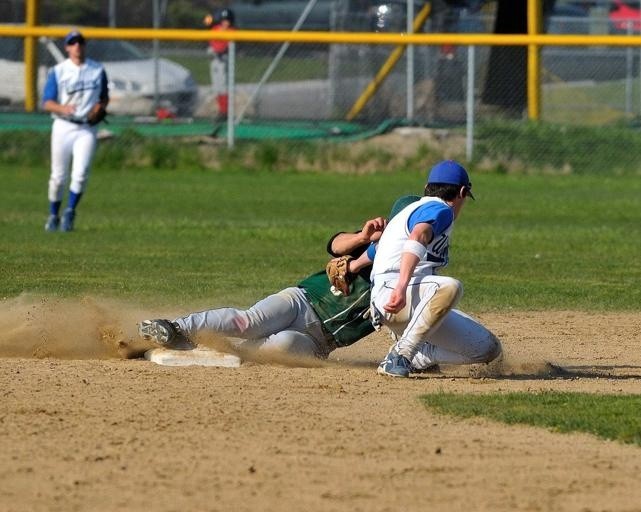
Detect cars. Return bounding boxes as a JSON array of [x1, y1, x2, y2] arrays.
[[0, 23, 198, 122], [211, 2, 333, 54], [333, 1, 640, 96]]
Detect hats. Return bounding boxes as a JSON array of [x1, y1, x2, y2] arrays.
[[64, 31, 84, 47], [427, 159, 475, 201]]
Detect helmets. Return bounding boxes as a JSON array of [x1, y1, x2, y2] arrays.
[[387, 194, 427, 234]]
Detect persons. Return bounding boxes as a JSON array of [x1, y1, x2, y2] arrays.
[[41, 30, 109, 232], [208, 10, 239, 121], [324, 160, 500, 379], [137, 195, 422, 363]]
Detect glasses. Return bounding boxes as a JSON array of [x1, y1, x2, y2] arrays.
[[67, 38, 84, 46]]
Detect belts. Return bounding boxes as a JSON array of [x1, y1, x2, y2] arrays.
[[59, 114, 97, 126]]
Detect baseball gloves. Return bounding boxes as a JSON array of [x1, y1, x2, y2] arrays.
[[326, 254, 356, 295], [87, 102, 107, 126]]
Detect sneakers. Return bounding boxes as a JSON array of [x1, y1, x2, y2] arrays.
[[410, 359, 446, 376], [136, 317, 200, 351], [60, 208, 76, 231], [43, 214, 59, 232], [377, 347, 410, 380]]
